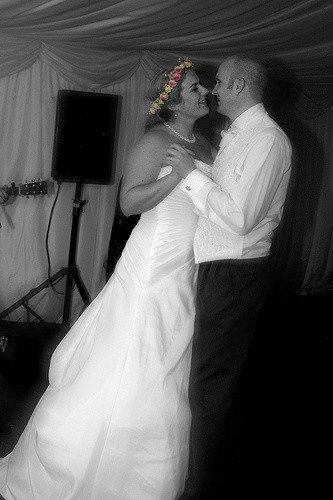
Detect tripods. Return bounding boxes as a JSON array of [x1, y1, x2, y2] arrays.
[[0, 182, 94, 329]]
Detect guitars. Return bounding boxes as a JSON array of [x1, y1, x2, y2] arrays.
[[0, 178, 47, 199]]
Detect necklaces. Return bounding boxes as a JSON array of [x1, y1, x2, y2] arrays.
[[164, 122, 196, 144]]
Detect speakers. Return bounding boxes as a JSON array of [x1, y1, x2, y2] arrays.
[[50, 89, 122, 187]]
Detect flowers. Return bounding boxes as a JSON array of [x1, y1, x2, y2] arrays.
[[148, 58, 192, 115]]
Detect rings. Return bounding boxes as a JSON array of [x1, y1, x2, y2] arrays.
[[170, 156, 173, 161]]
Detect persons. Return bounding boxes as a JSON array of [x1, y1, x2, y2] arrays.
[[160, 53, 293, 500], [0, 58, 218, 500]]
